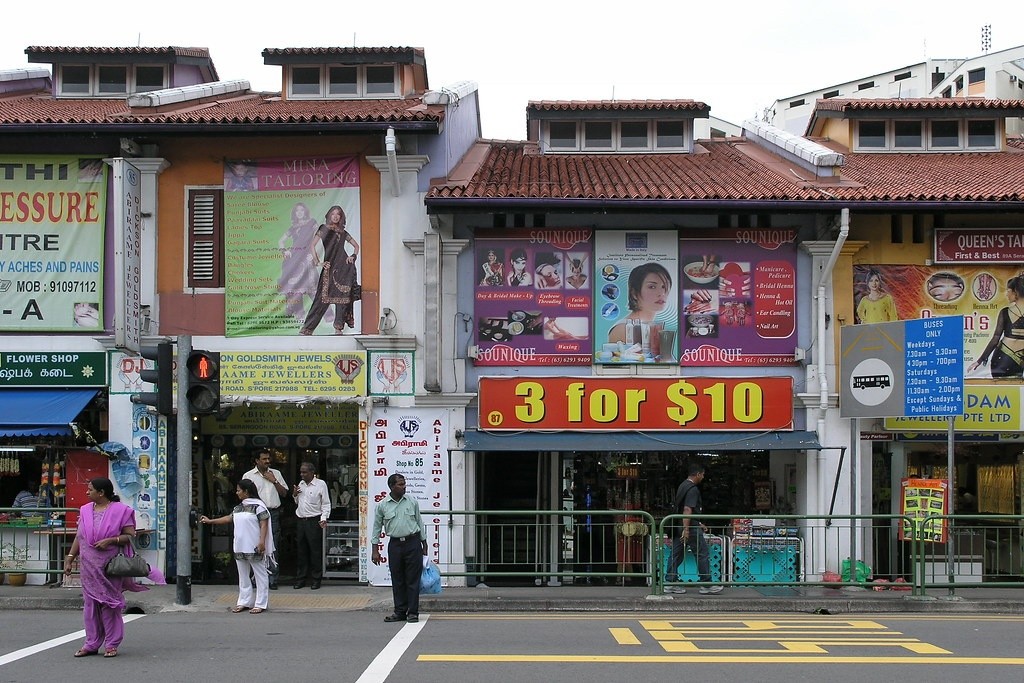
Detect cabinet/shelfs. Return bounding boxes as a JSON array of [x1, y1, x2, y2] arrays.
[[321, 520, 358, 580]]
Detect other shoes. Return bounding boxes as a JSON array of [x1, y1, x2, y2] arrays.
[[311, 584, 319, 589], [269, 583, 277, 590], [294, 585, 300, 589]]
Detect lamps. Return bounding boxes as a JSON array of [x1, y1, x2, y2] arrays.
[[0, 446, 36, 452], [794, 347, 806, 363], [467, 345, 480, 360], [139, 310, 151, 332], [378, 308, 391, 331]]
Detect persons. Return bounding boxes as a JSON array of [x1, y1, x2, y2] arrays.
[[968, 274, 1024, 377], [12, 479, 49, 517], [663, 464, 723, 593], [563, 479, 573, 498], [856, 271, 897, 323], [371, 474, 428, 623], [200, 479, 278, 614], [242, 450, 289, 590], [292, 462, 331, 590], [929, 275, 963, 301], [76, 303, 100, 328], [226, 164, 254, 191], [479, 250, 750, 343], [64, 478, 166, 658], [958, 487, 975, 513], [278, 204, 361, 335]]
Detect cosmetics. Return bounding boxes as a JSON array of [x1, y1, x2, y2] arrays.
[[626, 319, 676, 357]]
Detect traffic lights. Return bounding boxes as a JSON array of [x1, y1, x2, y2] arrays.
[[185, 349, 220, 414], [138, 344, 174, 416]]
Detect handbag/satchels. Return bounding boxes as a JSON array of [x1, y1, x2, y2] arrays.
[[106, 534, 151, 577], [665, 498, 684, 539], [419, 557, 441, 594]]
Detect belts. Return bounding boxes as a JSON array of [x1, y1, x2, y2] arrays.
[[390, 531, 419, 542], [298, 515, 321, 520]]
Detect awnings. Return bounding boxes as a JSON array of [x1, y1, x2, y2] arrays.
[[0, 388, 107, 453], [448, 430, 847, 528]]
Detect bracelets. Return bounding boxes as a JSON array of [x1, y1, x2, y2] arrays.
[[117, 538, 119, 543], [68, 554, 73, 557]]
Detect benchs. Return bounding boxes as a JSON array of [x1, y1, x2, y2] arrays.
[[910, 554, 983, 588]]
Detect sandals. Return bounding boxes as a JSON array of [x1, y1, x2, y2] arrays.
[[250, 607, 265, 613], [407, 613, 419, 623], [384, 614, 407, 622], [232, 605, 249, 613], [104, 651, 117, 657], [74, 647, 98, 657]]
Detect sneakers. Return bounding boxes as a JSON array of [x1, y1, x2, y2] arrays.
[[699, 586, 724, 594], [664, 586, 687, 594]]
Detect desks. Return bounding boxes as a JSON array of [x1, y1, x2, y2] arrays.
[[645, 535, 726, 587], [726, 533, 805, 586], [33, 528, 156, 588]]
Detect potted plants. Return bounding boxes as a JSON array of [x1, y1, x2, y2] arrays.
[[0, 541, 32, 586]]
